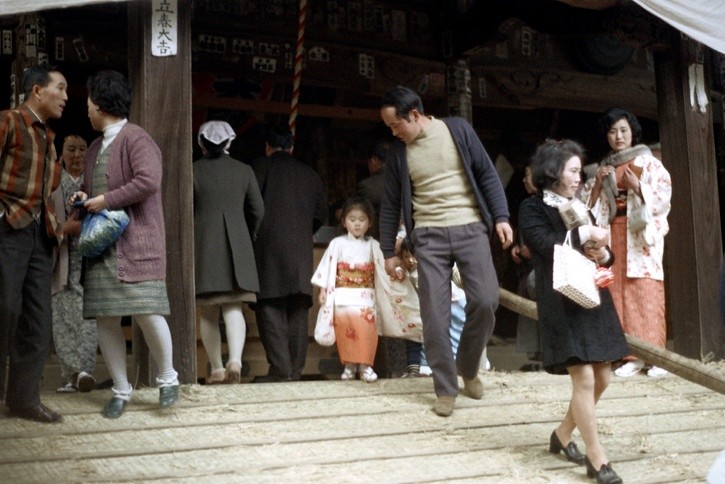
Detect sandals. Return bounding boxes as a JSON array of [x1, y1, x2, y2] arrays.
[[340, 363, 357, 380]]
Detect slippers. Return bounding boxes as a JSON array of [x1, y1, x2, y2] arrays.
[[647, 365, 669, 377], [357, 364, 377, 382], [614, 360, 645, 378]]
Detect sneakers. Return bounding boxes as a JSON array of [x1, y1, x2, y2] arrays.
[[76, 371, 96, 392], [56, 380, 79, 393]]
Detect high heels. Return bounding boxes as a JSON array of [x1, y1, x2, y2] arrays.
[[585, 455, 622, 484], [549, 429, 586, 464]]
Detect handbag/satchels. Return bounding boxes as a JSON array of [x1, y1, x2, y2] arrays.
[[553, 229, 601, 310]]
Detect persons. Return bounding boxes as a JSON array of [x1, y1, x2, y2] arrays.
[[515, 135, 634, 484], [511, 160, 575, 373], [358, 84, 517, 416], [71, 68, 180, 422], [187, 118, 266, 383], [0, 65, 71, 424], [308, 199, 398, 383], [46, 130, 104, 393], [575, 104, 673, 378], [237, 123, 332, 383]]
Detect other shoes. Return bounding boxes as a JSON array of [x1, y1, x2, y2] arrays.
[[208, 368, 226, 384], [225, 361, 243, 384], [402, 364, 420, 378]]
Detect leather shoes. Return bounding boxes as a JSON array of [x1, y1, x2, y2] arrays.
[[434, 396, 455, 416], [158, 385, 179, 407], [254, 374, 294, 382], [9, 402, 62, 422], [104, 398, 128, 418], [291, 372, 302, 382], [454, 359, 484, 399]]
